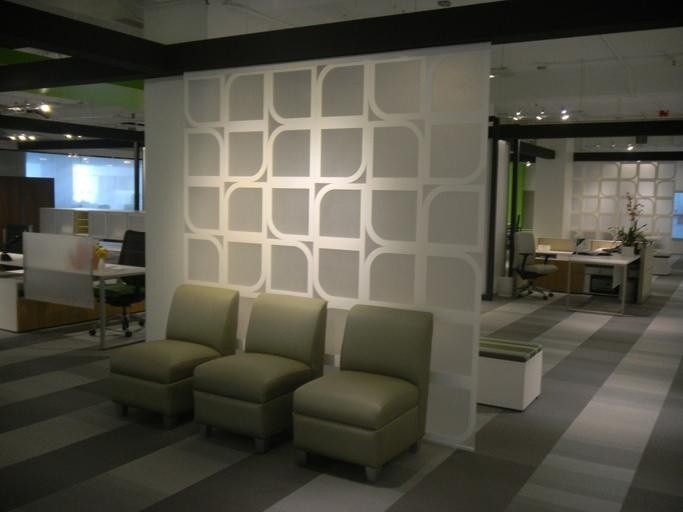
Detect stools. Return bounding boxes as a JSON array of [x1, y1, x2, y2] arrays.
[[651, 250, 672, 275], [476, 336, 543, 412]]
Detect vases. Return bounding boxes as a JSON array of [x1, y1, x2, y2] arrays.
[[98, 258, 105, 272], [622, 247, 634, 257]]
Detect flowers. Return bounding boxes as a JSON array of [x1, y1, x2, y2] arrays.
[[607, 191, 649, 255]]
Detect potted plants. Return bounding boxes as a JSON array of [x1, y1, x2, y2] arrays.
[[496, 214, 523, 298]]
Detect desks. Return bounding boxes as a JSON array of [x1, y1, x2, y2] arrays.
[[92, 263, 146, 350], [566, 255, 642, 317]]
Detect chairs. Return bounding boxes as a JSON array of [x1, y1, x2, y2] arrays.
[[89, 230, 145, 337], [110, 284, 239, 431], [292, 304, 433, 483], [512, 231, 559, 300], [192, 293, 327, 455]]
